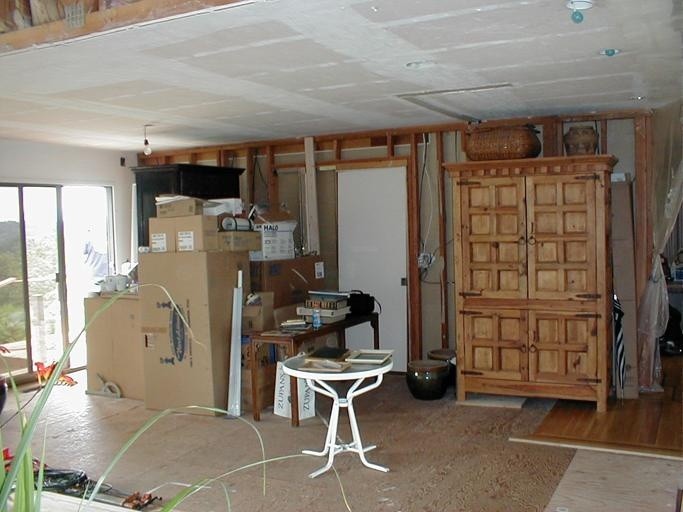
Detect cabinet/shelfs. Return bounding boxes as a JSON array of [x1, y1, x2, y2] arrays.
[[129, 163, 246, 248], [449, 156, 616, 413]]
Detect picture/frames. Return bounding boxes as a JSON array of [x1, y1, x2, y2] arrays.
[[305, 346, 351, 360], [297, 360, 351, 372], [345, 349, 395, 363]]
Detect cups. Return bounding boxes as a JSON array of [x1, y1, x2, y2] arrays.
[[105, 275, 130, 292]]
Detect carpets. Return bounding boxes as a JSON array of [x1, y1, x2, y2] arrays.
[[0, 368, 575, 512]]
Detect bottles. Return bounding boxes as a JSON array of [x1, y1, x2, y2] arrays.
[[312, 303, 320, 328]]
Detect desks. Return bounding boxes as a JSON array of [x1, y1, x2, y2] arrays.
[[281, 353, 393, 479], [5, 484, 140, 512], [250, 312, 379, 428]]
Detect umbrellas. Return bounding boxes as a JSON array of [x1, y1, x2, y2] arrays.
[[613, 289, 626, 407]]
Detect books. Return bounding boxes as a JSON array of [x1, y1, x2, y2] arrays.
[[260, 291, 351, 336], [308, 346, 350, 362]]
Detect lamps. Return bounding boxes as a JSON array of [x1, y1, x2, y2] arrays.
[[143, 124, 152, 156]]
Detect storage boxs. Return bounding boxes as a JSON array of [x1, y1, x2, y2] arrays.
[[84, 199, 329, 415]]
